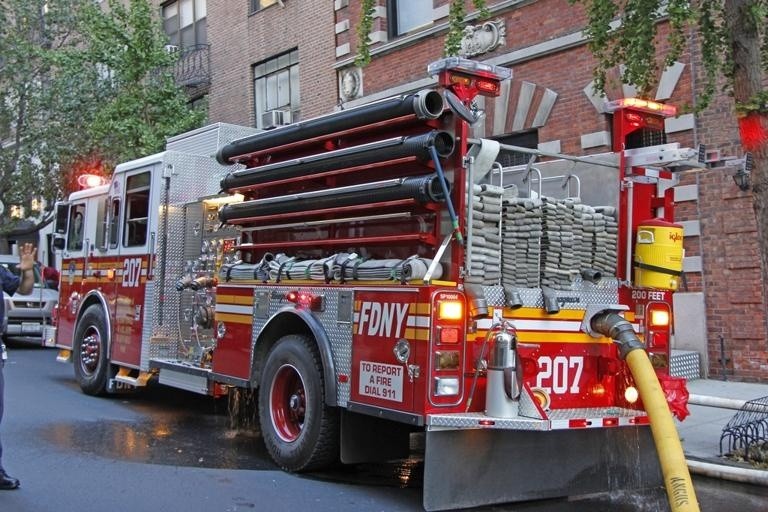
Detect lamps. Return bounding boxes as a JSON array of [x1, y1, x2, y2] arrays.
[[732, 167, 752, 192]]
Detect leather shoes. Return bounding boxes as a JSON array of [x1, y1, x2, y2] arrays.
[[0, 473, 20, 490]]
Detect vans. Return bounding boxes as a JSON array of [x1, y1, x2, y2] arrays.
[[0, 255, 58, 349]]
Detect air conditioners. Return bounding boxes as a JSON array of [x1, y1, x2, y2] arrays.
[[162, 44, 179, 61], [261, 110, 284, 129]]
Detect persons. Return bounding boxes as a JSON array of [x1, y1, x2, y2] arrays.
[[0, 243, 37, 491]]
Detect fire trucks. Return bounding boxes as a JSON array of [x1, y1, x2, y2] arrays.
[[44, 56, 688, 510]]
